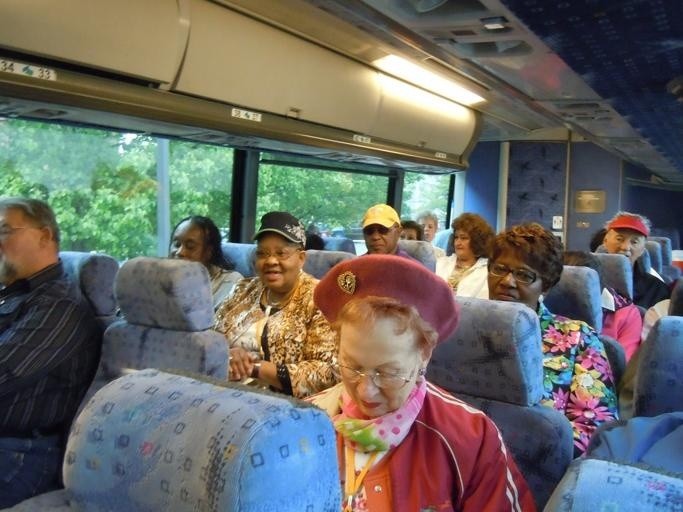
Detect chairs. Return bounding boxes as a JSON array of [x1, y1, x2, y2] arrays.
[[57, 251, 119, 333], [1, 369, 342, 511], [67, 258, 230, 437]]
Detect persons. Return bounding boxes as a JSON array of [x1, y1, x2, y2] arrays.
[[302, 202, 683, 508], [0, 200, 107, 510], [218, 211, 345, 401], [169, 218, 244, 315]]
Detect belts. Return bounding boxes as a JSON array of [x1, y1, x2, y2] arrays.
[[0, 424, 61, 438]]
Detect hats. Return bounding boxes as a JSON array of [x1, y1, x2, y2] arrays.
[[254, 211, 306, 247], [607, 218, 648, 238], [314, 254, 459, 343], [362, 204, 401, 229]]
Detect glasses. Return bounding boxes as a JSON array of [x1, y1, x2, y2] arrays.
[[366, 227, 387, 235], [257, 247, 298, 260], [489, 263, 542, 284], [328, 341, 418, 389]]
